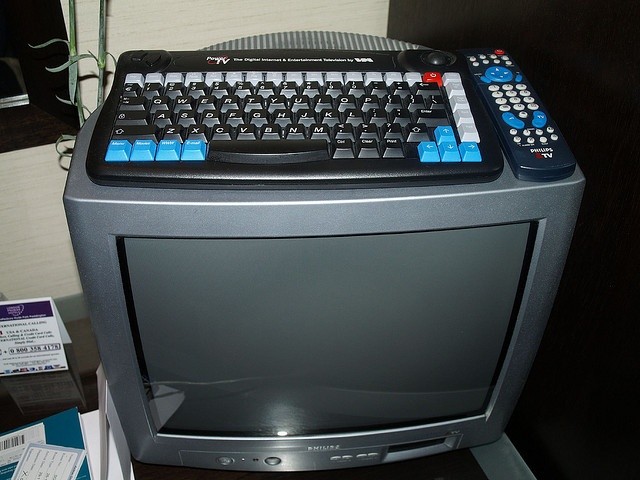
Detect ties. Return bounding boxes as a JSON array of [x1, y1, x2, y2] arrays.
[[63, 31, 587, 473]]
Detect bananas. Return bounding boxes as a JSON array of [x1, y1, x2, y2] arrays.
[[85, 50, 504, 190]]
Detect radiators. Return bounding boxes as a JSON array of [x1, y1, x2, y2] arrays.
[[462, 49, 577, 182]]
